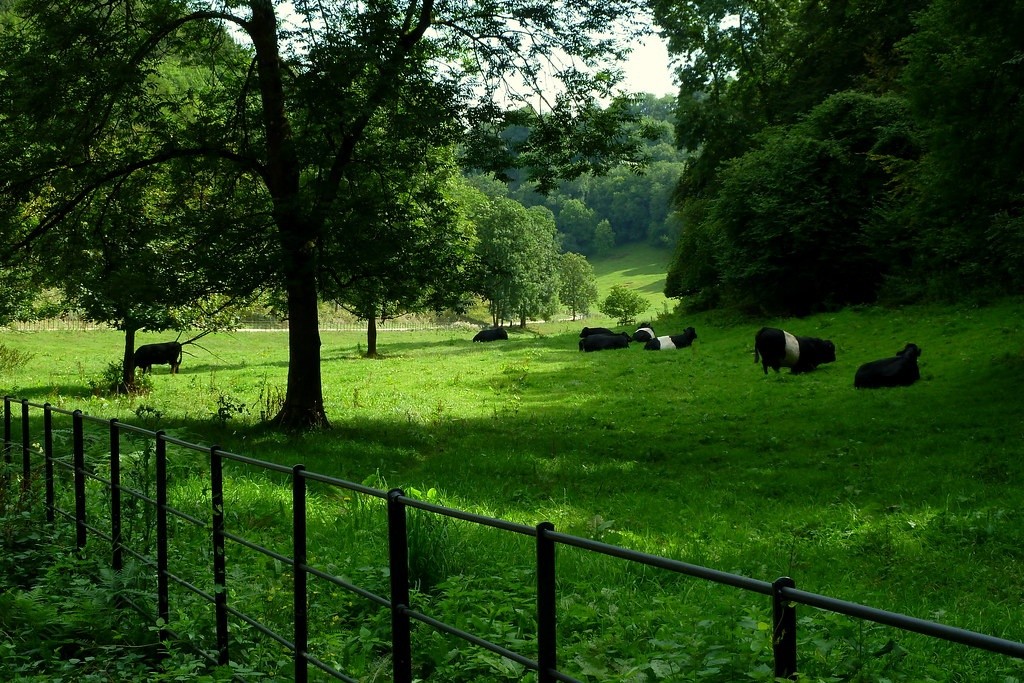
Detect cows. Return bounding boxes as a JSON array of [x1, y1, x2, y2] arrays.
[[578, 335, 628, 352], [580, 327, 616, 339], [855, 343, 921, 389], [473, 328, 507, 342], [632, 323, 655, 341], [645, 327, 698, 351], [754, 327, 836, 376], [133, 342, 182, 374]]
